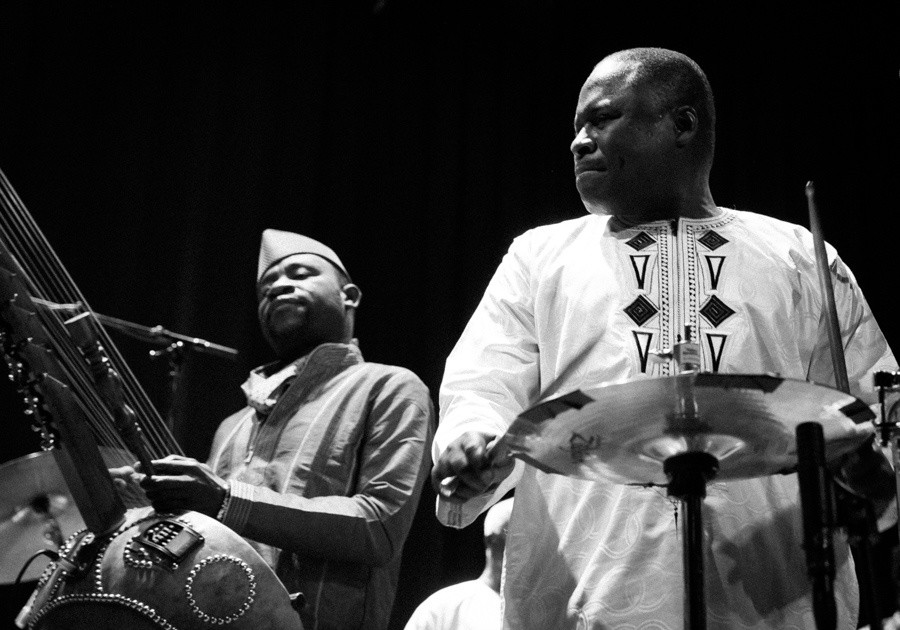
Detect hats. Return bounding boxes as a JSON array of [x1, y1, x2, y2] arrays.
[[257, 228, 353, 286]]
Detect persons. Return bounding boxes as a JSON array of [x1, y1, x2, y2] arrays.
[[105, 229, 434, 630], [403, 497, 514, 630], [430, 48, 900, 630]]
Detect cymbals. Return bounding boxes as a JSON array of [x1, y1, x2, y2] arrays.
[[503, 374, 877, 486]]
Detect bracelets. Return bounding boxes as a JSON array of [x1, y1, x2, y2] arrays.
[[217, 487, 229, 519]]
